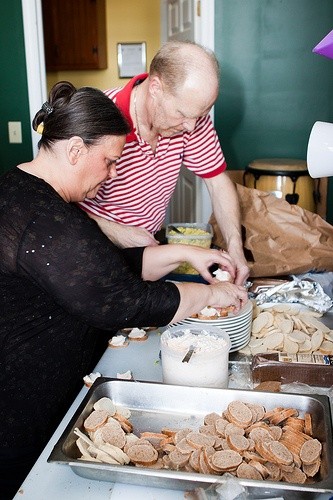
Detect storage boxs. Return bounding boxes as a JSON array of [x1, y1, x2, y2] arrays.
[[165, 223, 214, 275]]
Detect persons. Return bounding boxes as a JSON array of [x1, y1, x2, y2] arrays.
[[73, 40, 250, 286], [0, 80, 247, 500]]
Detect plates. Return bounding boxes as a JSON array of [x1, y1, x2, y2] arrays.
[[168, 299, 253, 353]]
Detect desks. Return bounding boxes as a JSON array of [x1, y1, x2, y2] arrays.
[[12, 280, 333, 500]]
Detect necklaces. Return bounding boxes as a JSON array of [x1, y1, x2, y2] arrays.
[[135, 84, 156, 153]]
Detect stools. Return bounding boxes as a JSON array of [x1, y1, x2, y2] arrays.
[[242, 158, 322, 214]]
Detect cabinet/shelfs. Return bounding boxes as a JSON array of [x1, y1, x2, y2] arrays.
[[42, 0, 108, 72]]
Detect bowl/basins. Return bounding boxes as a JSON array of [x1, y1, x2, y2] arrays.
[[160, 324, 231, 389]]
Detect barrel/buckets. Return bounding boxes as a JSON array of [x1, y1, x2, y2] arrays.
[[242, 158, 321, 214]]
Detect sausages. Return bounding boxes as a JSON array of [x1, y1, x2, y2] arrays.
[[242, 303, 333, 356], [107, 329, 149, 348], [81, 395, 323, 486], [190, 308, 233, 320]]
